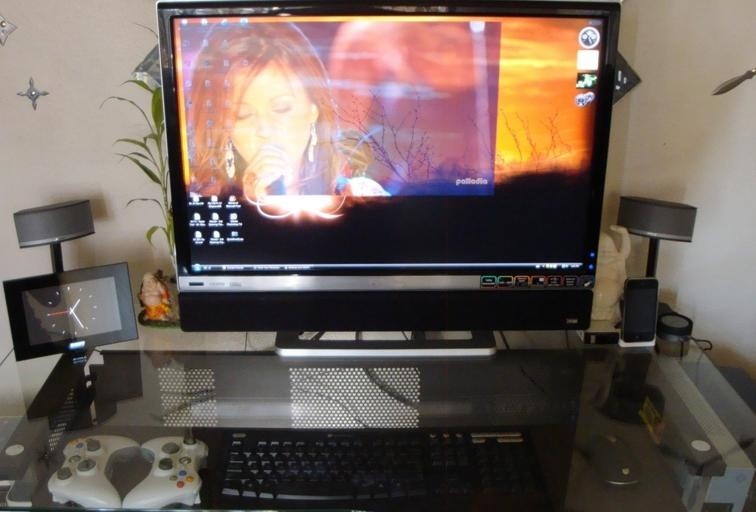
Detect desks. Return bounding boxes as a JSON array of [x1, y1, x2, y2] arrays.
[[0, 342, 756, 512]]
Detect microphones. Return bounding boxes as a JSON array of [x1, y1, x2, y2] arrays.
[[253, 122, 289, 197]]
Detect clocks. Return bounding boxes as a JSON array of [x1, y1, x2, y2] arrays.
[[2, 260, 140, 362]]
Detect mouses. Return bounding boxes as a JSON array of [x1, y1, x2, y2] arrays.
[[588, 433, 646, 488]]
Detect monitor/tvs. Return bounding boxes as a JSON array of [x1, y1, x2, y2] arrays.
[[153, 1, 624, 366]]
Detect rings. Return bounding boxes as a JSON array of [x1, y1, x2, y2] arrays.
[[248, 174, 262, 186]]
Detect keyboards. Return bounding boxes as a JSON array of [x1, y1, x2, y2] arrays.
[[202, 422, 561, 510]]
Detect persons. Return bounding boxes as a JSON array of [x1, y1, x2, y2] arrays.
[[591, 224, 633, 320], [139, 272, 174, 321], [182, 22, 393, 201]]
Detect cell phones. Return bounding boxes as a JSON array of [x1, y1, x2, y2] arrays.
[[605, 350, 653, 420], [622, 277, 659, 346]]
